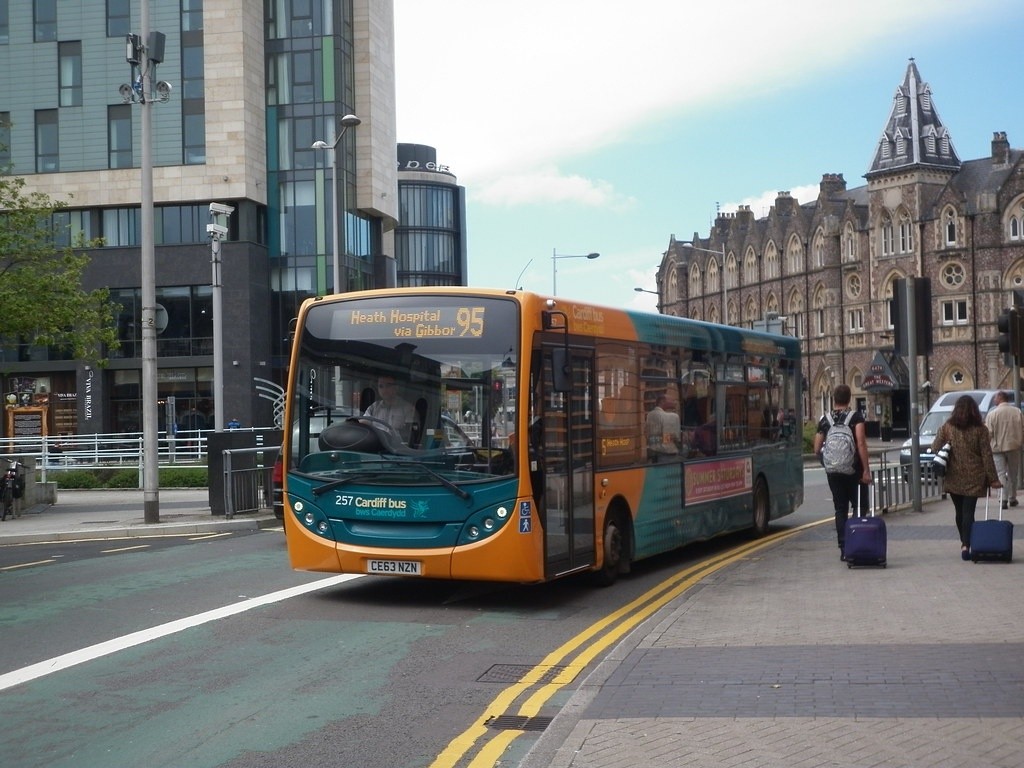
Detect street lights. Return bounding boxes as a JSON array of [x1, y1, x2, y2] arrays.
[[635, 280, 663, 313], [682, 241, 729, 326], [552, 249, 599, 296], [312, 114, 362, 413]]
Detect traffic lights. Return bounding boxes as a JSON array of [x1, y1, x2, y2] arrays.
[[997, 311, 1017, 353], [493, 380, 503, 404]]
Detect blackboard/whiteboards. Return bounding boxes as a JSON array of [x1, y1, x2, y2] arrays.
[[8, 406, 47, 453]]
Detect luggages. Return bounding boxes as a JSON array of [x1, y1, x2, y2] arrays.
[[843, 478, 887, 569], [970, 485, 1013, 564]]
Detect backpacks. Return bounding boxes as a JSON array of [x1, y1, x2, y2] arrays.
[[822, 408, 857, 475]]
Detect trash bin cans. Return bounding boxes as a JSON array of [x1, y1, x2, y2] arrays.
[[881, 426, 892, 441]]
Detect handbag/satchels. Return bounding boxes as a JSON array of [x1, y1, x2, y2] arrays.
[[930, 423, 952, 477]]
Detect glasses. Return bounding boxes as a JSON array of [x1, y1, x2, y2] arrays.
[[378, 383, 394, 389]]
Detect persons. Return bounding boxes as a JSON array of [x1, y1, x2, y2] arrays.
[[984, 391, 1024, 509], [931, 395, 1001, 560], [692, 397, 736, 458], [777, 407, 795, 437], [814, 385, 873, 561], [358, 373, 420, 447], [645, 394, 681, 453]]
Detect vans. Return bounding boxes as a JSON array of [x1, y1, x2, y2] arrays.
[[900, 389, 1024, 482]]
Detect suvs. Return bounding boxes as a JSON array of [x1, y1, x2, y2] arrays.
[[272, 406, 474, 534]]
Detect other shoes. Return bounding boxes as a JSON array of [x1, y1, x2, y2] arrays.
[[999, 500, 1008, 509], [1010, 499, 1018, 506], [962, 550, 971, 560]]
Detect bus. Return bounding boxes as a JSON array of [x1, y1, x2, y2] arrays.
[[281, 285, 805, 585]]
